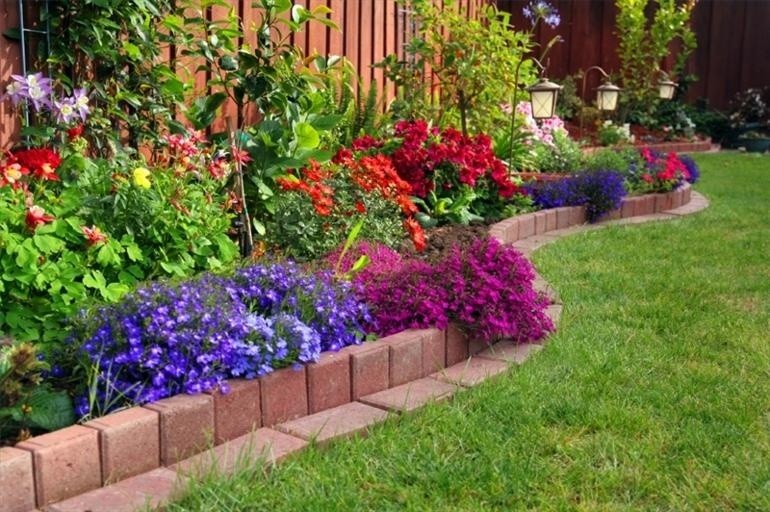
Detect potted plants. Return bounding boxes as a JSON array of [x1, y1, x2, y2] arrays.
[[728, 86, 770, 153]]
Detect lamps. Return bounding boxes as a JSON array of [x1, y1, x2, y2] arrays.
[[508, 55, 560, 179], [645, 70, 679, 142], [579, 65, 620, 148]]
